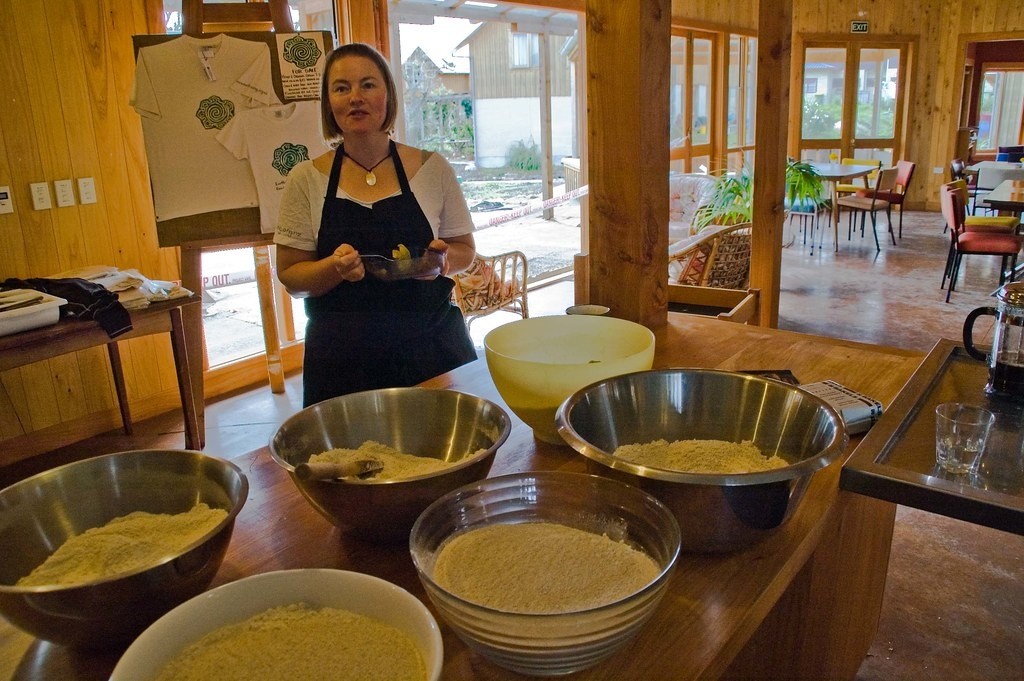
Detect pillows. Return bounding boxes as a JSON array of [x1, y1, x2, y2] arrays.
[[996, 145, 1024, 162]]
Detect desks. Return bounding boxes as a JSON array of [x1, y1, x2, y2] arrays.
[[983, 178, 1024, 241], [0, 310, 930, 680], [808, 162, 880, 245], [0, 287, 201, 452]]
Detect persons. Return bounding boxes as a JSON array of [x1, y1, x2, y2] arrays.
[[273, 43, 476, 409]]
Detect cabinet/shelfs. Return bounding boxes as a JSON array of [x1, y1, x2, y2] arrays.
[[956, 126, 978, 165]]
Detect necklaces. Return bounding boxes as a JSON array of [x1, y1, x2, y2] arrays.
[[344, 153, 391, 186]]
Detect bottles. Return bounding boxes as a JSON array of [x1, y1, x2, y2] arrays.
[[829, 152, 838, 174]]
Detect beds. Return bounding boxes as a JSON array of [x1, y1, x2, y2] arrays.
[[971, 160, 1024, 189]]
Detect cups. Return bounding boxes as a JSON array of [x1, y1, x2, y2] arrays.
[[933, 402, 996, 476]]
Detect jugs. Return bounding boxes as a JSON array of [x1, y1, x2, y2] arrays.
[[962, 281, 1024, 409]]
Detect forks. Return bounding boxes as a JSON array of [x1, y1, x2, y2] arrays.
[[334, 253, 395, 262]]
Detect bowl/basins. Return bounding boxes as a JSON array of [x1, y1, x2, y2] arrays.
[[553, 367, 845, 559], [268, 386, 512, 545], [564, 303, 612, 316], [482, 313, 656, 448], [108, 567, 445, 681], [365, 247, 446, 280], [407, 467, 684, 676], [0, 448, 248, 653]]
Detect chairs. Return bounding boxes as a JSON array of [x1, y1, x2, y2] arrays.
[[837, 165, 896, 251], [784, 157, 829, 255], [667, 222, 752, 289], [669, 172, 726, 243], [444, 250, 528, 331], [853, 160, 916, 238], [940, 158, 1021, 302], [828, 157, 881, 231]]
